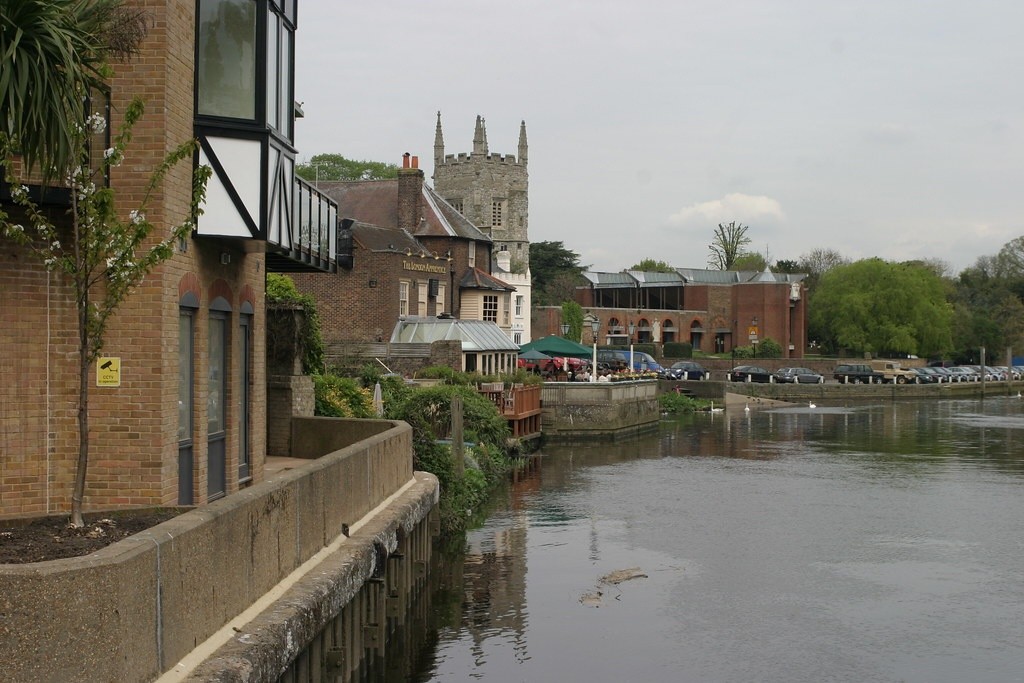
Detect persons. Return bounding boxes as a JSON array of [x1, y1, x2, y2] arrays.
[[534, 363, 626, 381]]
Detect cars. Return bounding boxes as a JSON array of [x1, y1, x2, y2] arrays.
[[726, 366, 778, 383], [900, 365, 1024, 384], [518, 356, 602, 374], [665, 361, 705, 381], [774, 367, 825, 384]]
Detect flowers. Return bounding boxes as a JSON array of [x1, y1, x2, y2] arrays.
[[610, 372, 658, 378]]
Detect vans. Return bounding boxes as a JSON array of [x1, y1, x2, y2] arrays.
[[597, 349, 677, 380]]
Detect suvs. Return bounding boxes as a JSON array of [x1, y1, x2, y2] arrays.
[[597, 350, 628, 370], [832, 364, 883, 384], [872, 360, 917, 384]]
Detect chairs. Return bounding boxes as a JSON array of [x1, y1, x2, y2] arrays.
[[482, 382, 524, 410]]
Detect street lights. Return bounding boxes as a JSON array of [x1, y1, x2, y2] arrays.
[[628, 320, 637, 373], [592, 315, 599, 382], [561, 320, 570, 371]]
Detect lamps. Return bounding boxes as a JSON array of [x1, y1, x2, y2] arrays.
[[442, 253, 453, 262], [388, 243, 394, 249], [430, 250, 439, 260], [417, 249, 426, 258], [403, 246, 412, 256]]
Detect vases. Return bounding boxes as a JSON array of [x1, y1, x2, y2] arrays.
[[641, 376, 647, 379], [634, 377, 640, 380], [619, 378, 624, 381], [611, 378, 617, 381], [647, 376, 650, 379], [626, 377, 632, 380]]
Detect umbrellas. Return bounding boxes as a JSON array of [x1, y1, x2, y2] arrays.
[[518, 335, 593, 374]]
